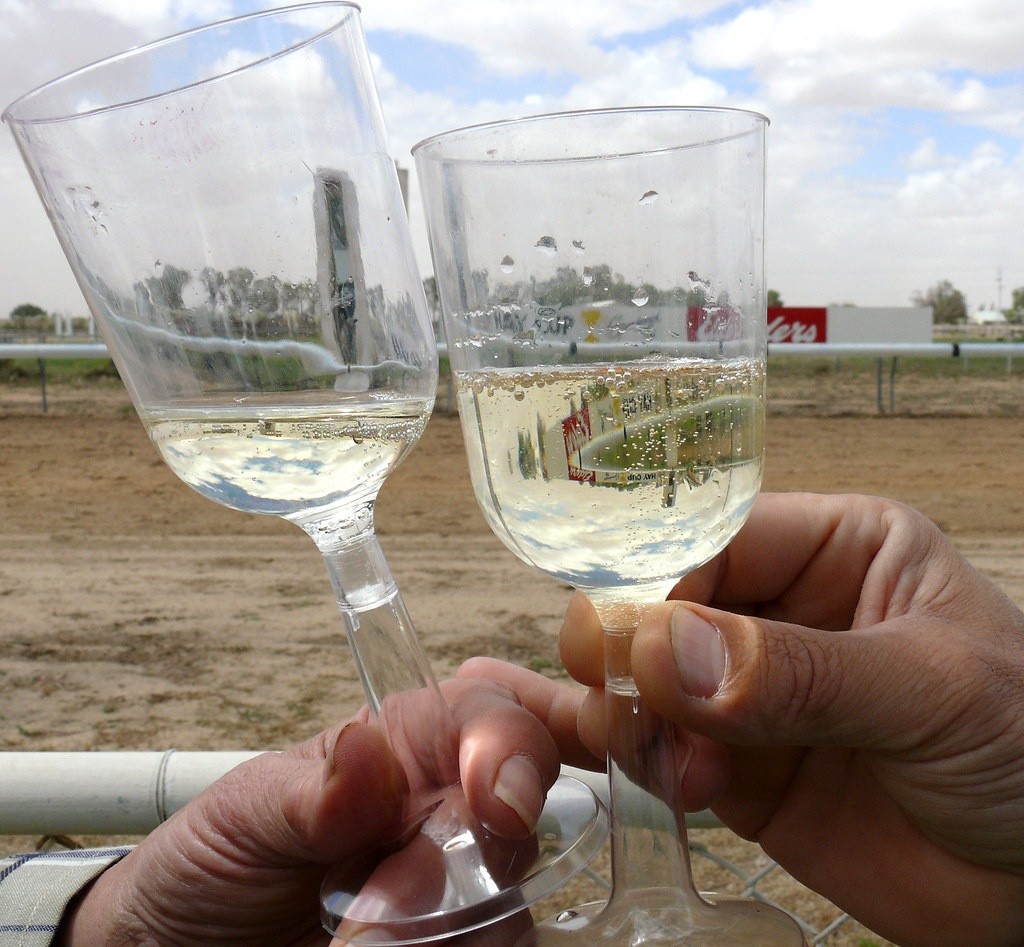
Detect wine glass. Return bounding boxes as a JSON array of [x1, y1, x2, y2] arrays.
[[0, 0, 609, 947], [408, 106, 811, 947]]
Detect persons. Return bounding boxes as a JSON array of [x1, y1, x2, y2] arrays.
[[453, 491, 1024, 946], [0, 677, 561, 947]]
